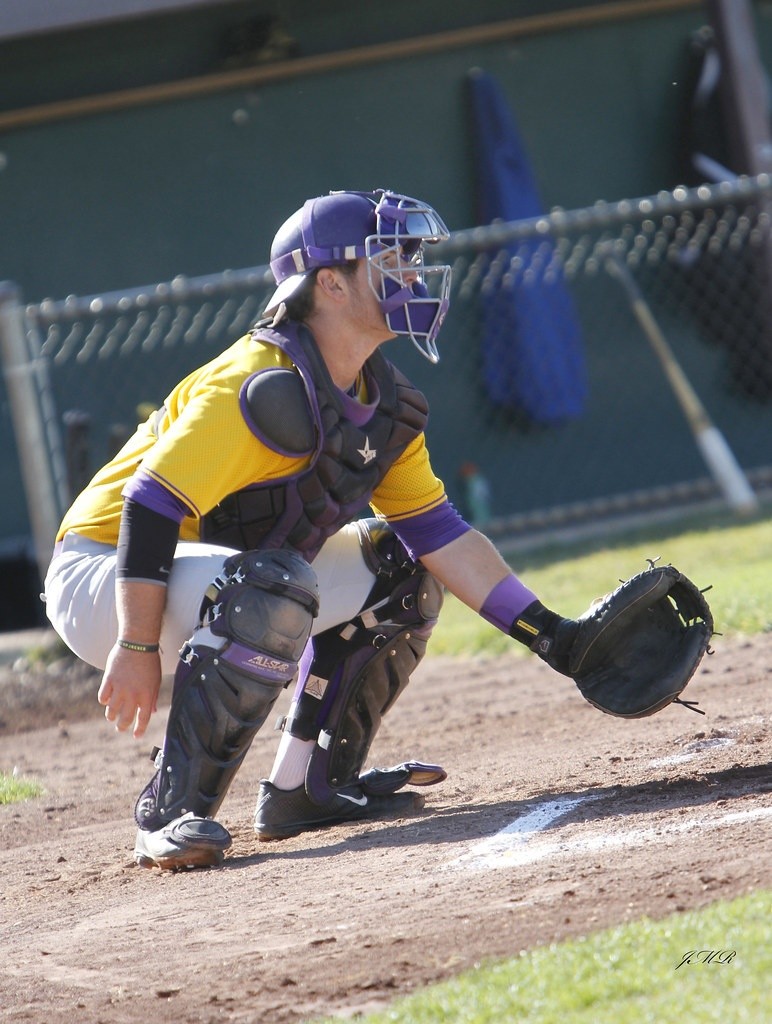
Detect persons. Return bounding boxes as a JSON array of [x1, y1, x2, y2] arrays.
[[39, 190, 715, 870]]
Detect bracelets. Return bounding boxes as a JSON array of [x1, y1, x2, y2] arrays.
[[118, 640, 159, 652]]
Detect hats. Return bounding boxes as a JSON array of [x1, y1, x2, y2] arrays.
[[259, 190, 423, 312]]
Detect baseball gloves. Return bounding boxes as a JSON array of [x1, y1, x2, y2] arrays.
[[566, 566, 715, 719]]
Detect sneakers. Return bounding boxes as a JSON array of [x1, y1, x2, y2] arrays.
[[135, 829, 225, 870], [255, 779, 426, 841]]
